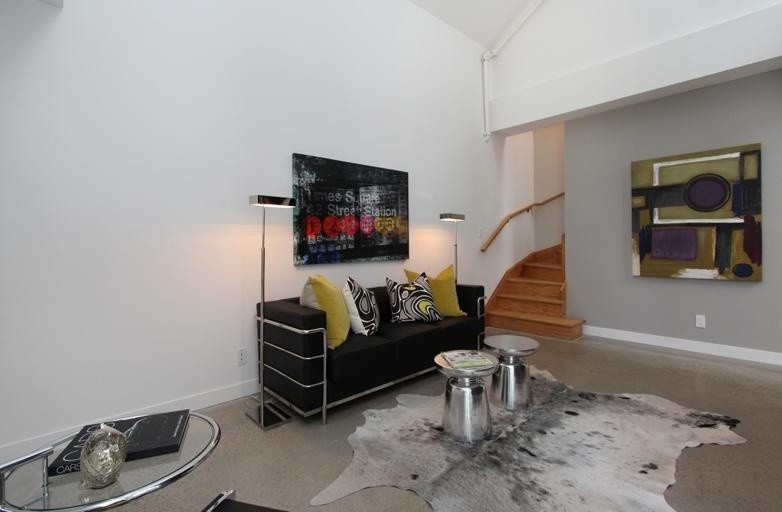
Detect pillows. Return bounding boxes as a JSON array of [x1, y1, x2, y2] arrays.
[[299, 264, 470, 352]]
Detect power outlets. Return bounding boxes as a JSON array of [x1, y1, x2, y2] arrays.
[[236, 348, 247, 367]]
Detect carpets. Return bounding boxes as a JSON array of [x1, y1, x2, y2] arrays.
[[309, 363, 750, 511]]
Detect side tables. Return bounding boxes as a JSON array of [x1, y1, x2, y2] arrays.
[[433, 334, 542, 443]]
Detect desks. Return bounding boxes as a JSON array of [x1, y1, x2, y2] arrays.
[[1, 407, 222, 512]]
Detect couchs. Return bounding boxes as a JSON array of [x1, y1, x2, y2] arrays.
[[256, 283, 487, 426]]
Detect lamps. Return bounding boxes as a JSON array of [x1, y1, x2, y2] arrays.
[[245, 192, 296, 431], [439, 212, 466, 284]]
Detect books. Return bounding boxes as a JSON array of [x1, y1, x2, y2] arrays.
[[47, 405, 190, 477], [442, 351, 493, 368]]
